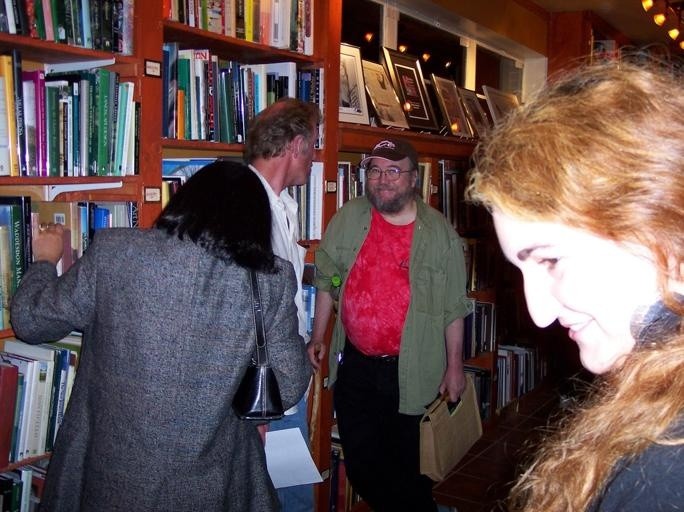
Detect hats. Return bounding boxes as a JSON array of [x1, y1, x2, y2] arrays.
[[360, 138, 417, 169]]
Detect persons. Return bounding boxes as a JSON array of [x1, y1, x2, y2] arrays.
[[10, 160, 311, 511], [465, 57, 684, 512], [244, 99, 313, 511], [308, 139, 470, 512]]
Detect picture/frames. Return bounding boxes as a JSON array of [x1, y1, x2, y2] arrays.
[[339, 42, 370, 126], [431, 73, 474, 137], [383, 46, 441, 132], [459, 86, 493, 138], [483, 85, 520, 125], [363, 60, 411, 130]]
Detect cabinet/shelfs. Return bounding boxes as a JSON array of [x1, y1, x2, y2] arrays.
[[0, 0, 547, 512]]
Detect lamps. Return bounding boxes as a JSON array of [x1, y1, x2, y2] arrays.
[[639, 0, 684, 50]]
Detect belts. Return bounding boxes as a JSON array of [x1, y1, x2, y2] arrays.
[[368, 356, 398, 368]]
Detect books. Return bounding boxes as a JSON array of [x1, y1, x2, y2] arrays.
[[302, 264, 317, 335], [1, 50, 141, 177], [164, 0, 314, 55], [329, 410, 364, 511], [461, 238, 548, 420], [0, 195, 139, 330], [161, 157, 222, 209], [286, 160, 368, 241], [414, 159, 479, 228], [0, 332, 83, 512], [1, 0, 134, 54], [163, 41, 324, 149]]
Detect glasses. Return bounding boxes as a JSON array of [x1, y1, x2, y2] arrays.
[[363, 164, 411, 180]]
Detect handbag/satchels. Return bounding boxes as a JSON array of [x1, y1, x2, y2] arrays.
[[420, 372, 483, 482], [232, 271, 284, 421]]
